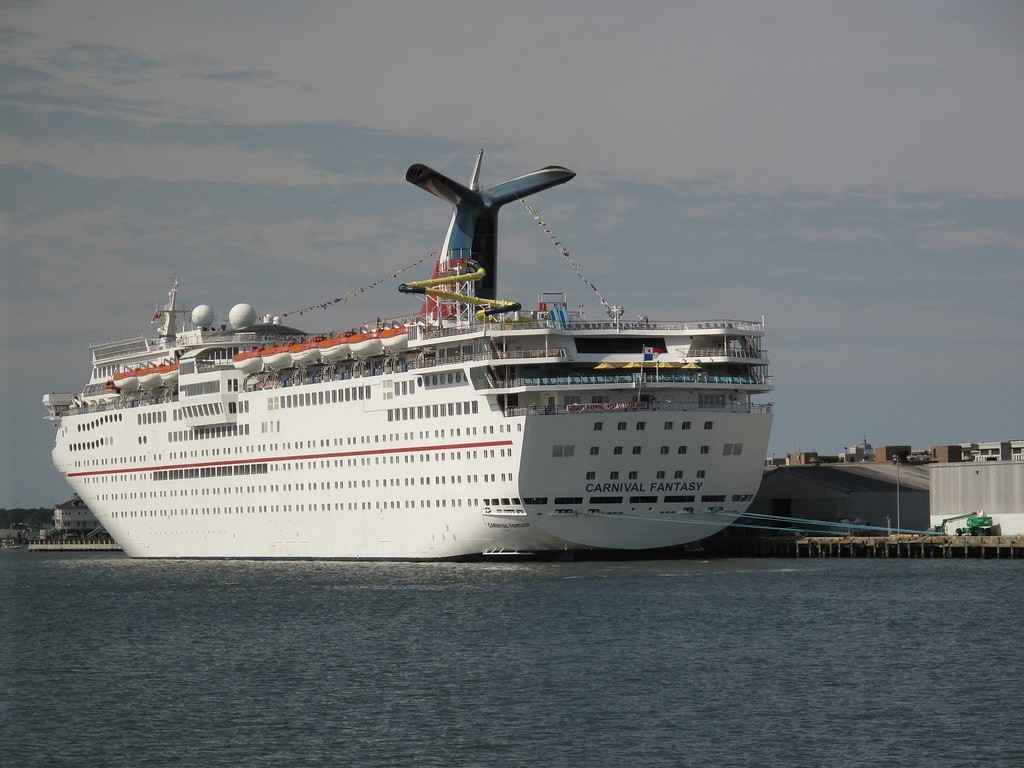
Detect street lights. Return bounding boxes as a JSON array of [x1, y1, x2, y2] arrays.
[[891, 454, 901, 542]]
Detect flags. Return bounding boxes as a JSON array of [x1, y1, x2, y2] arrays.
[[644, 347, 663, 361]]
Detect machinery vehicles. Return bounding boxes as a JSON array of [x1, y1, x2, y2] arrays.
[[933, 510, 993, 536]]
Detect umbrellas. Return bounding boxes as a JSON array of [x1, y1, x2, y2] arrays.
[[594, 362, 702, 376]]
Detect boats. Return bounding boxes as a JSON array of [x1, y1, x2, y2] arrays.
[[288, 342, 320, 365], [113, 371, 139, 389], [231, 350, 262, 373], [137, 366, 162, 386], [380, 328, 408, 351], [317, 336, 349, 361], [349, 333, 382, 357], [261, 345, 292, 369], [158, 364, 178, 380]]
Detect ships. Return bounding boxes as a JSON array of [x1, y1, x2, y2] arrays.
[[40, 146, 775, 563]]
